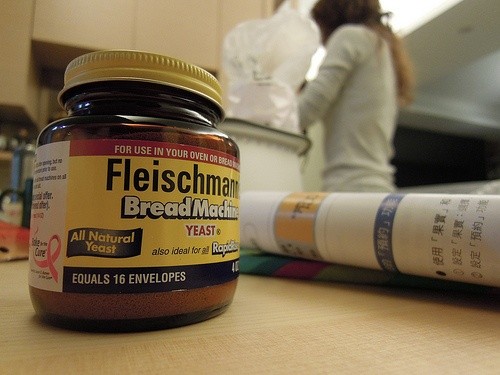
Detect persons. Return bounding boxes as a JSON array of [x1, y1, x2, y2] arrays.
[[297, 0, 401, 195]]
